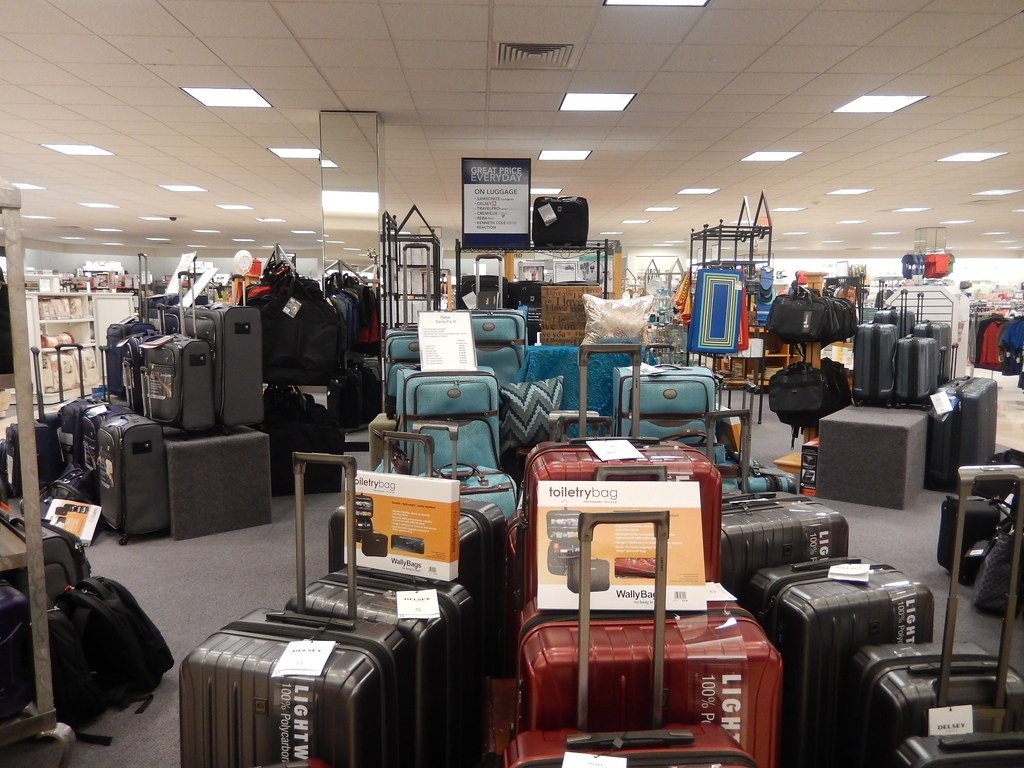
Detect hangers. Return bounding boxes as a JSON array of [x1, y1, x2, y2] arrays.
[[343, 269, 350, 278], [261, 252, 304, 298]]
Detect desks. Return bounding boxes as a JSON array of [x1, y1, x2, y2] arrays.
[[816, 404, 929, 510], [369, 412, 397, 471], [162, 425, 273, 540], [515, 344, 657, 438]]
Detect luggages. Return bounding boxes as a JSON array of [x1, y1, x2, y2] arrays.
[[911, 291, 951, 383], [852, 274, 899, 407], [176, 342, 1024, 768], [895, 290, 938, 409], [0, 251, 268, 547], [873, 280, 915, 338], [383, 242, 432, 396], [455, 255, 529, 390], [931, 343, 998, 491], [0, 579, 35, 721]]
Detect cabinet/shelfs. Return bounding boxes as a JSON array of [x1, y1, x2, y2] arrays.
[[397, 204, 452, 327], [455, 238, 622, 345], [0, 179, 58, 745], [5, 291, 134, 404], [707, 327, 806, 390]]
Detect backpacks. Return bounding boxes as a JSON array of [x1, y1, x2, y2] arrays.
[[10, 499, 91, 588], [22, 590, 113, 745], [56, 576, 173, 713]]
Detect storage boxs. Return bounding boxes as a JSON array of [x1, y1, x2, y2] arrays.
[[45, 499, 102, 546], [537, 480, 708, 612], [800, 437, 820, 498], [344, 469, 459, 581], [715, 402, 742, 451]]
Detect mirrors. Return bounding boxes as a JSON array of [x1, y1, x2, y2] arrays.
[[321, 190, 381, 288]]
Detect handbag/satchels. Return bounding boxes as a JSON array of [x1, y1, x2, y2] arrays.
[[972, 449, 1024, 500], [764, 278, 862, 432], [532, 197, 589, 246], [237, 242, 382, 495], [975, 500, 1024, 615]]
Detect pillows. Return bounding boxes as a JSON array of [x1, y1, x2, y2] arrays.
[[500, 376, 564, 458], [581, 294, 654, 344]]
